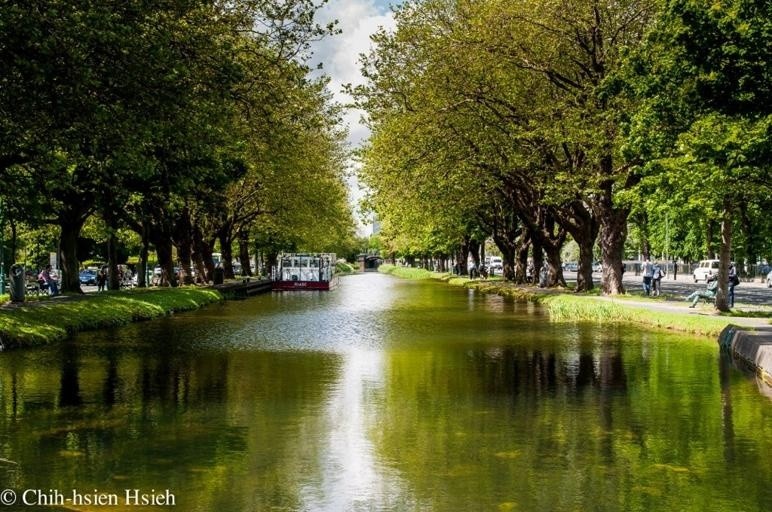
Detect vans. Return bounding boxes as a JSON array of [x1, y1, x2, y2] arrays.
[[691, 260, 719, 283]]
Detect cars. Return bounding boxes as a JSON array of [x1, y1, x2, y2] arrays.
[[78, 249, 269, 291], [444, 257, 606, 275]]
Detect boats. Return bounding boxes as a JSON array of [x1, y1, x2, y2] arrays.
[[269, 249, 340, 292]]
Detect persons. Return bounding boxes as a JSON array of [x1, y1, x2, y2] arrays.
[[652, 262, 663, 296], [642, 260, 652, 295], [35, 264, 60, 299], [684, 274, 718, 308], [727, 263, 738, 308], [672, 261, 678, 280], [97, 267, 109, 292]]
